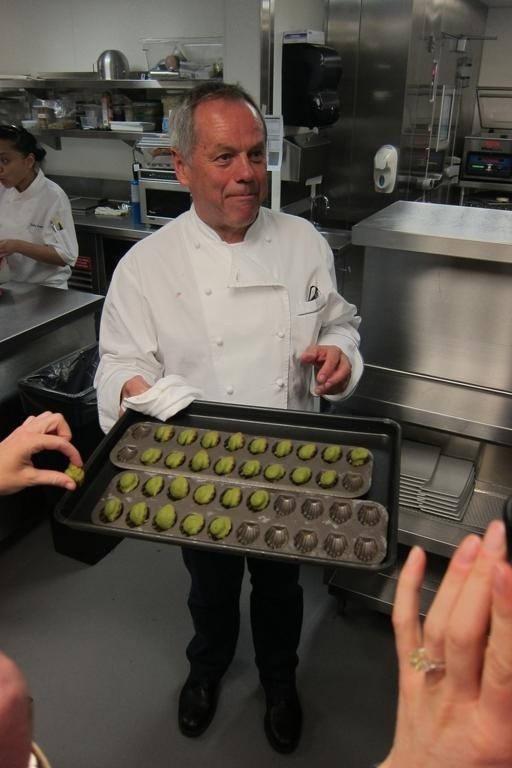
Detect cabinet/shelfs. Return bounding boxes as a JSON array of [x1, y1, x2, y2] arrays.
[[326, 372, 511, 633], [0, 69, 222, 147]]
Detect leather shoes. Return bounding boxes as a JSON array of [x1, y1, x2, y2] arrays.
[[258, 669, 303, 755], [178, 668, 223, 738]]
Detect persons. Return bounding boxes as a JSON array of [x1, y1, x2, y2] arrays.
[[90, 77, 362, 755], [368, 517, 512, 767], [1, 116, 80, 290], [0, 409, 85, 499]]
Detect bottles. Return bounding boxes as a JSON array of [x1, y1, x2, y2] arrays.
[[101, 90, 114, 130]]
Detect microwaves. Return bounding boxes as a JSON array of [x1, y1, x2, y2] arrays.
[[137, 166, 194, 230]]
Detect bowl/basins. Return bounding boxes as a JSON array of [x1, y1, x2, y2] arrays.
[[81, 116, 98, 130]]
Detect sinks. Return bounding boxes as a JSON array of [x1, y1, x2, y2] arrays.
[[297, 211, 358, 235]]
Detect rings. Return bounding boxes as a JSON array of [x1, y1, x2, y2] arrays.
[[409, 648, 444, 676]]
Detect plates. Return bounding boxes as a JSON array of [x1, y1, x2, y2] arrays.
[[399, 421, 485, 522], [108, 119, 157, 131]]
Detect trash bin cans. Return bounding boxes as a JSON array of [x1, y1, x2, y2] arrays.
[[17, 343, 136, 566]]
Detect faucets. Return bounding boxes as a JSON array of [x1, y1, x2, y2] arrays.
[[309, 195, 330, 222]]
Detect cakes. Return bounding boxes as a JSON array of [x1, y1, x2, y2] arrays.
[[66, 464, 86, 485], [105, 425, 370, 540]]
